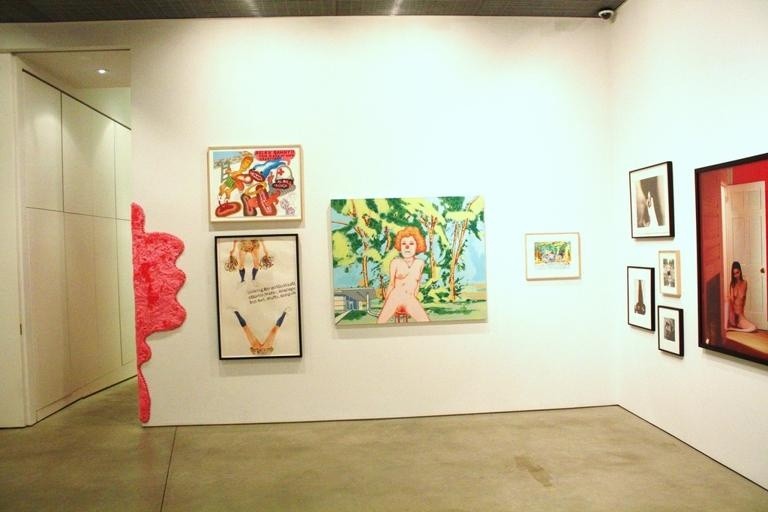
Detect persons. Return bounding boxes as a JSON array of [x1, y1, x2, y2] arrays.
[[664, 264, 673, 286], [726, 261, 758, 332]]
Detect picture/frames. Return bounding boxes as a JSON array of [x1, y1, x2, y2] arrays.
[[657, 304, 688, 357], [694, 147, 768, 368], [212, 229, 305, 364], [625, 264, 656, 336], [520, 231, 583, 282], [202, 142, 309, 226], [627, 159, 677, 242], [656, 249, 682, 298]]
